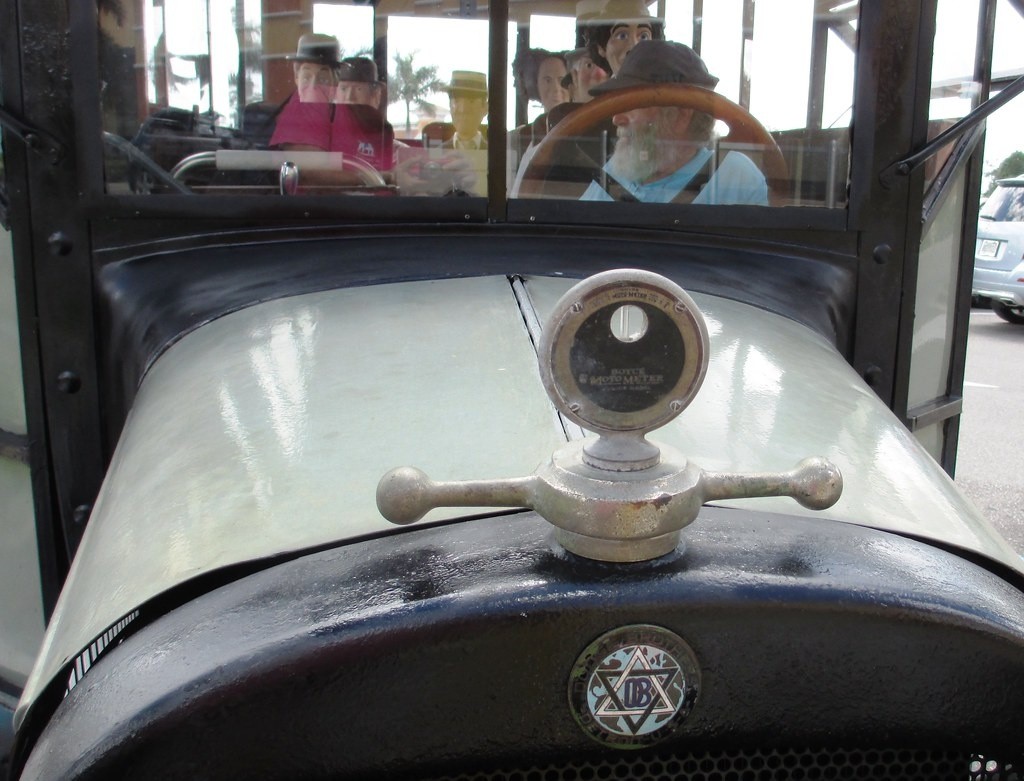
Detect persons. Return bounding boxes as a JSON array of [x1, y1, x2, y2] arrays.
[[579, 41, 769, 207], [335, 57, 411, 166], [507, 48, 572, 170], [438, 70, 488, 150], [286, 34, 355, 101], [585, 0, 666, 78], [564, 12, 611, 103]]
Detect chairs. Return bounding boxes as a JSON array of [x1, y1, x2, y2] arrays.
[[422, 98, 620, 182]]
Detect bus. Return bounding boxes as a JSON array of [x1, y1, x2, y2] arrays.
[[0, 0, 1024, 781]]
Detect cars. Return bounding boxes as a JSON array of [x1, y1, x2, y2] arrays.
[[970, 173, 1024, 324]]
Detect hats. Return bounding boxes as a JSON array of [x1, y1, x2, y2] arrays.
[[585, 40, 719, 92]]
